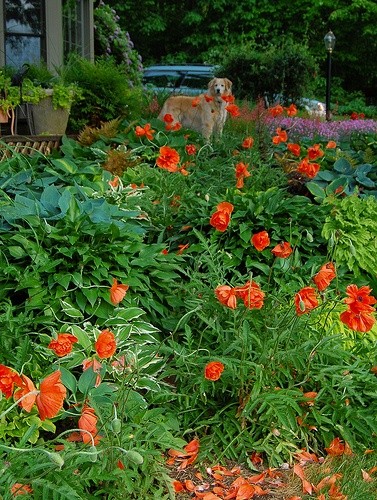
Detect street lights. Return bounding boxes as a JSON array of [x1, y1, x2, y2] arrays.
[[324, 31, 336, 121]]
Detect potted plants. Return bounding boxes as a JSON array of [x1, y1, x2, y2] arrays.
[[0, 64, 76, 136]]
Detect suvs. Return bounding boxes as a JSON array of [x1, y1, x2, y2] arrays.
[[139, 62, 231, 98]]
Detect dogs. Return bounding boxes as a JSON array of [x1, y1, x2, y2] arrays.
[[157, 77, 233, 149]]
